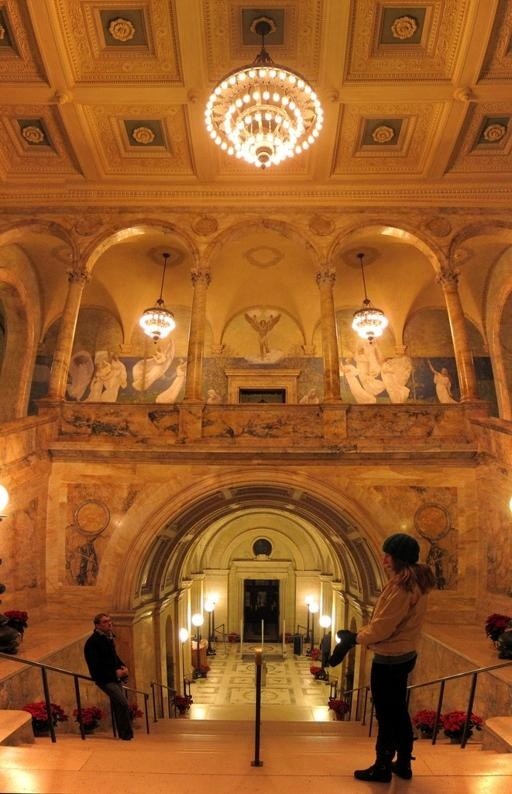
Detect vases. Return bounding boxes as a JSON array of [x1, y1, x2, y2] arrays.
[[333, 712, 347, 721], [31, 724, 51, 737], [312, 655, 318, 659], [419, 725, 472, 745], [82, 726, 95, 735], [314, 672, 319, 679]]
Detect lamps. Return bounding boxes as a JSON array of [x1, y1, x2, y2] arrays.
[[349, 251, 388, 346], [200, 11, 323, 172], [140, 249, 178, 344]]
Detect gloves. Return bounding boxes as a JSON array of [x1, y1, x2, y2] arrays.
[[328, 628, 359, 668]]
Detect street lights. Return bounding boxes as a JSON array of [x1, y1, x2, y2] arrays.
[[319, 614, 331, 670], [303, 593, 314, 643], [308, 601, 320, 650], [204, 600, 216, 656], [179, 627, 189, 696], [207, 592, 220, 642], [192, 613, 204, 679]]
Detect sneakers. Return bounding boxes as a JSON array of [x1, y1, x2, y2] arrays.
[[353, 760, 413, 784]]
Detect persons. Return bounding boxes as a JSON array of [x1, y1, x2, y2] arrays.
[[205, 389, 220, 403], [426, 358, 458, 405], [84, 613, 134, 740], [340, 338, 413, 405], [328, 533, 438, 785], [319, 631, 332, 668], [66, 336, 189, 404], [299, 388, 320, 404], [244, 312, 283, 361]]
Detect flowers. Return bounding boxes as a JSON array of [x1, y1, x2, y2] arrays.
[[412, 706, 484, 738], [481, 612, 511, 646], [175, 696, 193, 713], [5, 609, 29, 632], [307, 648, 321, 657], [326, 700, 351, 714], [26, 701, 67, 731], [125, 704, 143, 720], [310, 665, 321, 674], [74, 707, 104, 726], [198, 665, 210, 678]]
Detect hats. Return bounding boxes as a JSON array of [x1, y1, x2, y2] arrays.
[[380, 532, 421, 566]]
